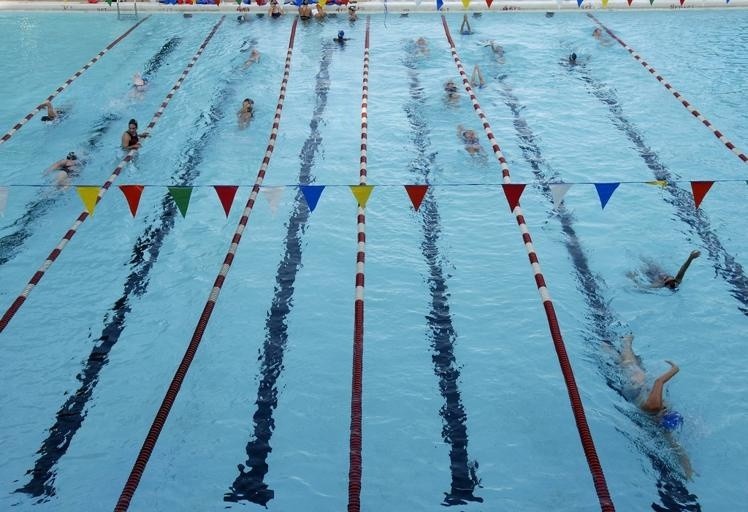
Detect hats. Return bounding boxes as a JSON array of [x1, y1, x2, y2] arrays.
[[662, 412, 684, 431], [338, 31, 344, 37], [303, 0, 308, 5], [244, 99, 253, 105], [569, 53, 577, 61], [348, 6, 357, 11], [129, 119, 138, 126], [67, 151, 78, 159], [665, 279, 679, 289], [41, 116, 51, 121]]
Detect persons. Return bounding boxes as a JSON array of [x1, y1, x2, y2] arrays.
[[237, 5, 250, 22], [559, 53, 592, 71], [268, 1, 327, 18], [592, 29, 610, 45], [129, 72, 150, 100], [122, 119, 149, 151], [417, 13, 504, 144], [333, 29, 348, 41], [42, 150, 83, 188], [237, 99, 255, 118], [348, 5, 357, 21], [603, 335, 693, 481], [624, 250, 702, 290], [36, 98, 56, 125]]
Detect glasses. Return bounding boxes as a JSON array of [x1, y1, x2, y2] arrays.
[[663, 276, 674, 281]]
